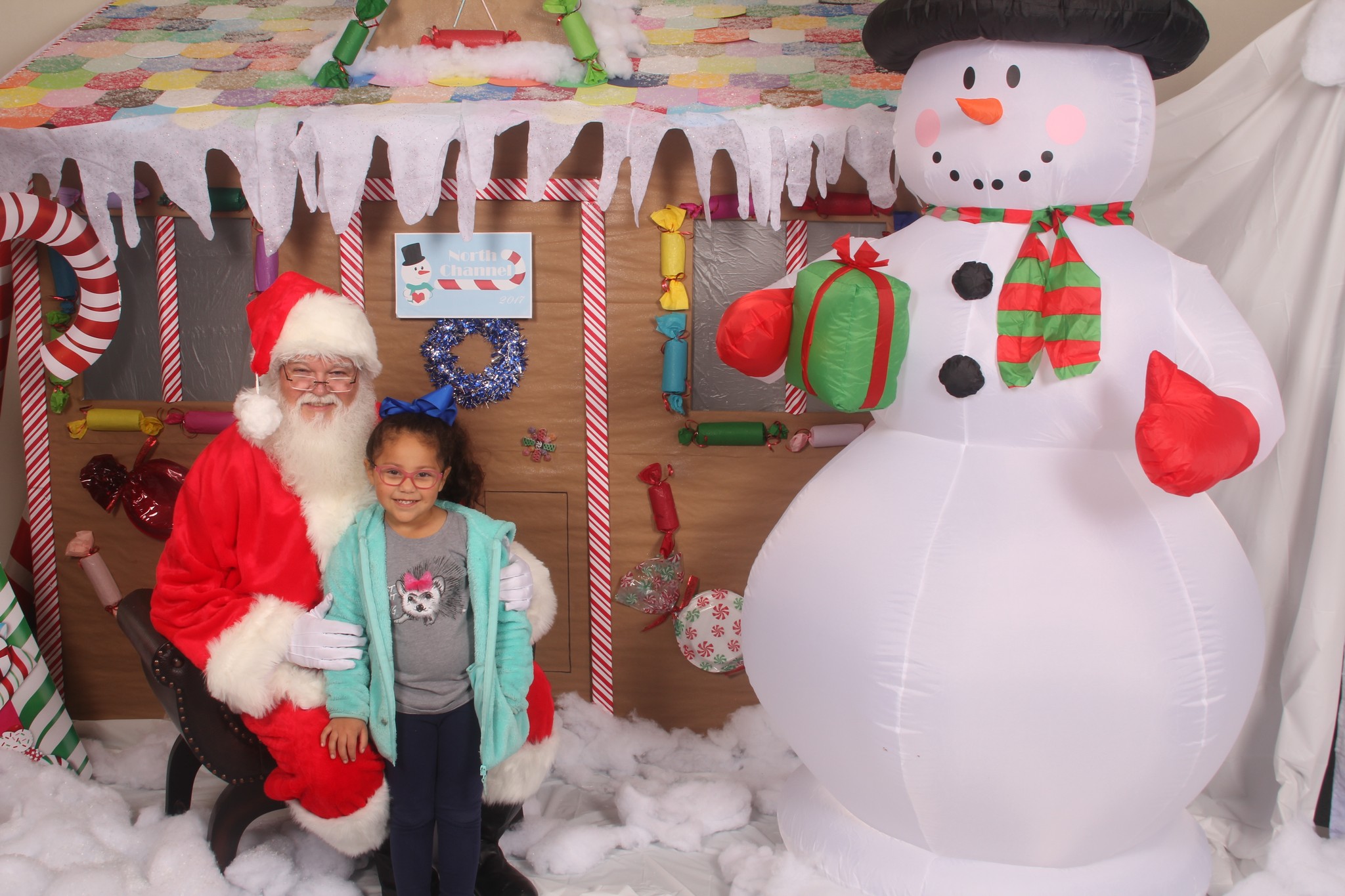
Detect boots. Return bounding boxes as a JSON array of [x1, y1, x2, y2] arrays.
[[476, 803, 539, 896]]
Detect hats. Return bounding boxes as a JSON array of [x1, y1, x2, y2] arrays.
[[234, 270, 382, 440]]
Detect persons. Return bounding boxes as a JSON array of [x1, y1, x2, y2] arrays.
[[310, 406, 543, 896], [147, 266, 557, 896], [705, 0, 1294, 896]]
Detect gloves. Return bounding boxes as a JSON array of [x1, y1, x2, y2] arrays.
[[499, 535, 534, 611], [285, 593, 367, 670]]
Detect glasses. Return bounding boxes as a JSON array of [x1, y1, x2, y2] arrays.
[[369, 460, 445, 489], [282, 363, 358, 392]]
